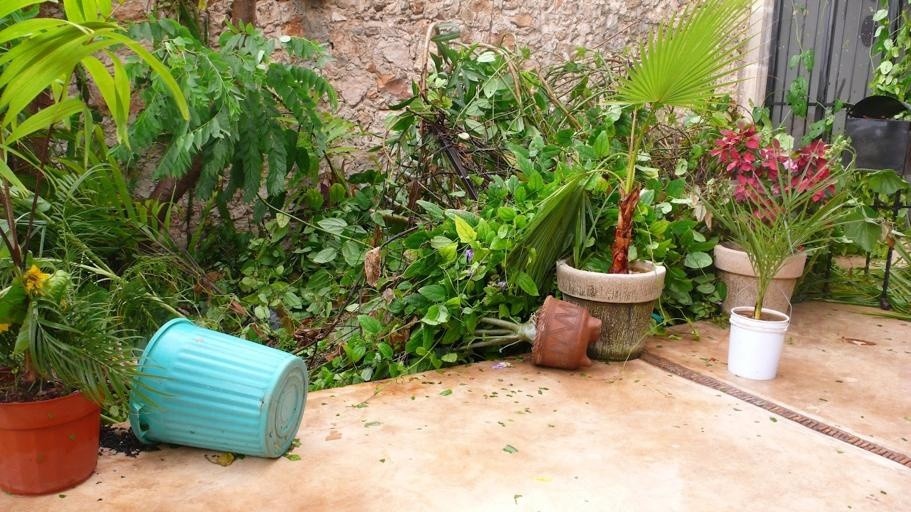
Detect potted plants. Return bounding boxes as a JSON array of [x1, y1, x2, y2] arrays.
[[29, 164, 308, 459], [686, 123, 870, 381], [510, 0, 765, 361], [395, 282, 603, 369]]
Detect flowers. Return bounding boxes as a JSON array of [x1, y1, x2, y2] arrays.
[[0, 264, 74, 384], [710, 122, 836, 230]]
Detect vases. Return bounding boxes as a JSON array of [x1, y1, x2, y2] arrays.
[[713, 244, 807, 320], [0, 365, 108, 496]]
